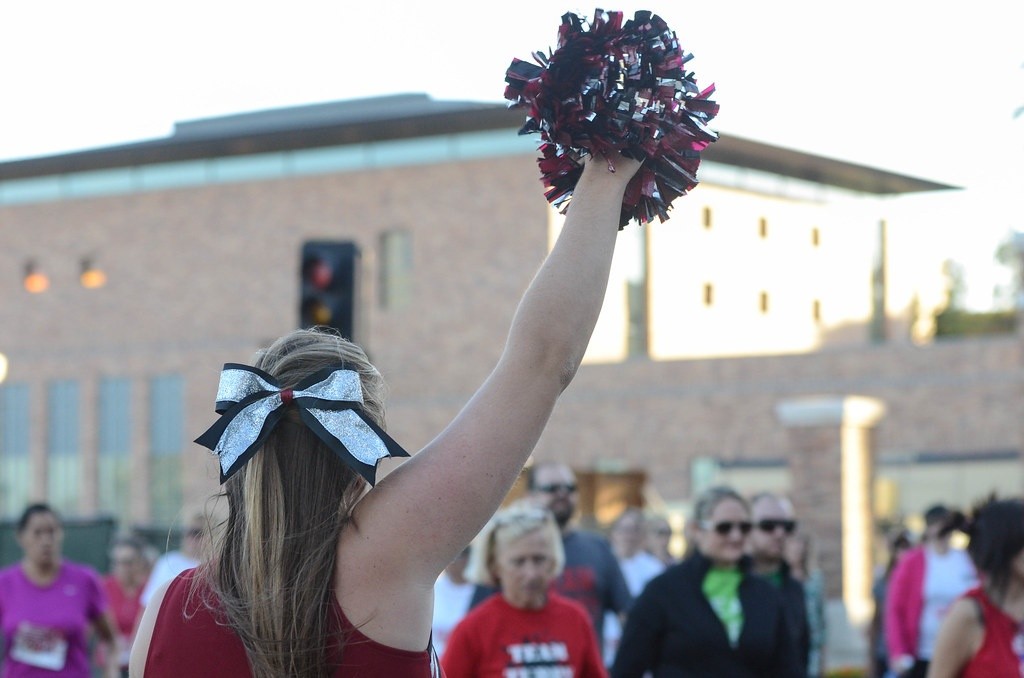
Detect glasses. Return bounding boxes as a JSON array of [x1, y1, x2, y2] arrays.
[[697, 522, 751, 535], [757, 519, 797, 534], [536, 483, 578, 493]]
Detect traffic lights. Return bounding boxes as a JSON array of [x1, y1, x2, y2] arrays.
[[299, 240, 357, 343]]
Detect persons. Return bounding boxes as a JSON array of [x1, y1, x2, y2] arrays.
[[928, 496, 1024, 677], [601, 512, 677, 666], [103, 511, 212, 678], [749, 493, 830, 678], [436, 510, 610, 678], [0, 505, 121, 678], [128, 90, 659, 678], [430, 546, 477, 678], [871, 536, 912, 678], [469, 462, 631, 660], [611, 488, 800, 678], [884, 503, 978, 678]]
